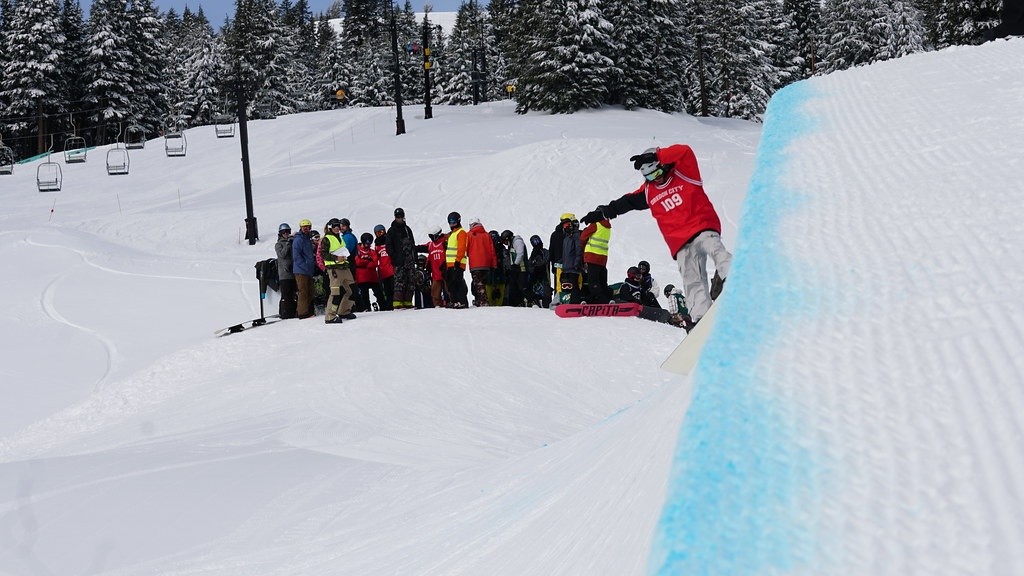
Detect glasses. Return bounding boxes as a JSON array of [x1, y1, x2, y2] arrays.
[[643, 168, 663, 181], [448, 219, 456, 224], [561, 283, 573, 290], [281, 230, 289, 234], [635, 277, 640, 280], [375, 230, 384, 236], [563, 222, 571, 228], [330, 223, 340, 228], [312, 235, 320, 240]]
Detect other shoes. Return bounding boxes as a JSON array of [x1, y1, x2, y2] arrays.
[[686, 322, 697, 334], [394, 299, 489, 311], [325, 317, 342, 324], [339, 312, 356, 320], [710, 269, 726, 300]]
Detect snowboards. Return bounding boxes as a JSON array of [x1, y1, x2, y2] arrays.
[[554, 300, 642, 317], [617, 300, 670, 323], [216, 314, 281, 339]]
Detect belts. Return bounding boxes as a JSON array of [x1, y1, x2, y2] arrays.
[[687, 228, 717, 243]]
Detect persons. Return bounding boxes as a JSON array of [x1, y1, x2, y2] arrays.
[[465, 222, 497, 307], [549, 212, 616, 308], [253, 208, 418, 324], [415, 211, 469, 310], [489, 229, 553, 308], [578, 143, 733, 324], [335, 88, 348, 107], [614, 259, 661, 308], [663, 284, 693, 331]]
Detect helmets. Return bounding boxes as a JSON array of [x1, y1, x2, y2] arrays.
[[627, 266, 640, 278], [639, 147, 663, 175], [663, 284, 675, 298], [595, 205, 605, 210], [277, 206, 579, 266], [638, 261, 650, 274]]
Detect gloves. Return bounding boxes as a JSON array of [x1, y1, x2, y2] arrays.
[[580, 210, 604, 225], [453, 261, 460, 271], [630, 152, 658, 170]]
